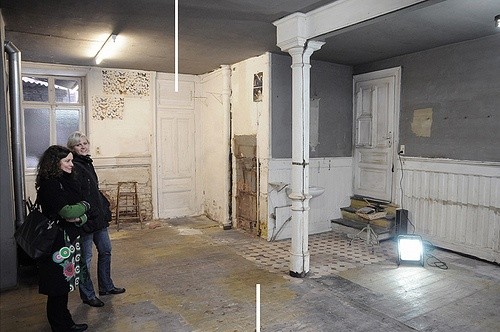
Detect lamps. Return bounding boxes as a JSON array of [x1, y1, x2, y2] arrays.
[[397, 234, 425, 268], [92, 34, 116, 65]]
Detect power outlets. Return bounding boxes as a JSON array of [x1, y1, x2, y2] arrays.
[[400, 145, 406, 155]]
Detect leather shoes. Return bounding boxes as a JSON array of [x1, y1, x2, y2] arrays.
[[83, 296, 104, 307], [99, 286, 126, 296], [69, 324, 88, 332]]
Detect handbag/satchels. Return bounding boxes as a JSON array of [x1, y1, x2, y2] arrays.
[[13, 188, 66, 259]]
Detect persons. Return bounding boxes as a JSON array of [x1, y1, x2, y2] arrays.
[[35, 145, 91, 332], [64, 131, 125, 307]]
